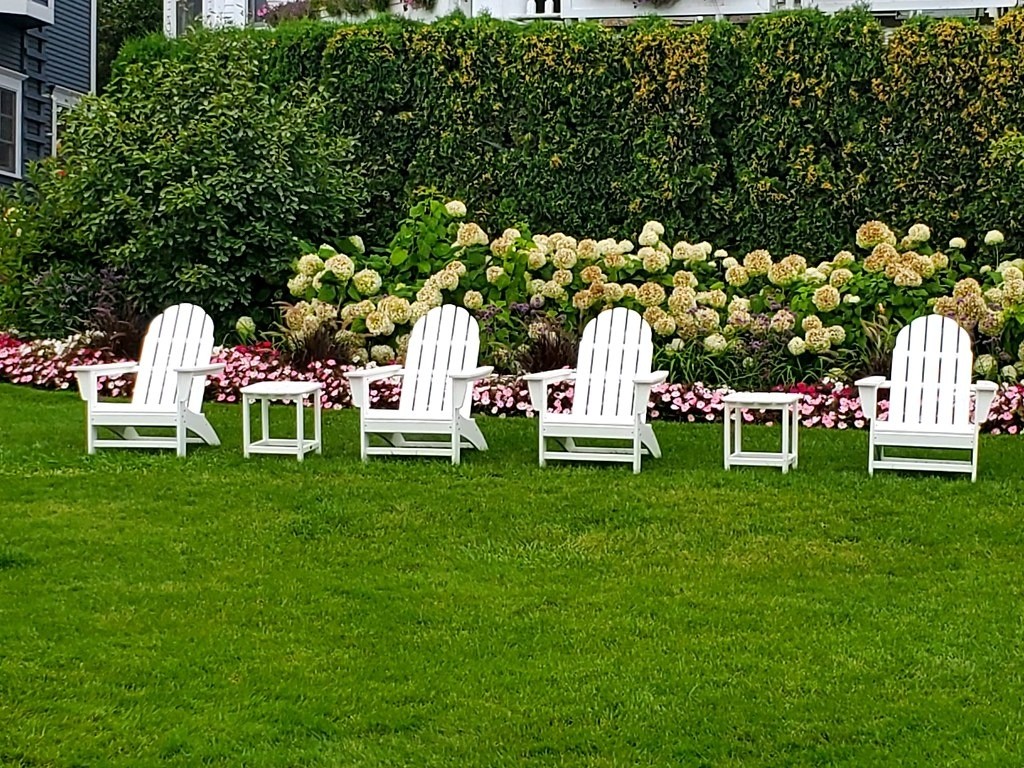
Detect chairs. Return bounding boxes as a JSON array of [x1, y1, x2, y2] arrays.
[[344, 304, 494, 467], [66, 303, 225, 461], [522, 307, 669, 474], [855, 314, 997, 482]]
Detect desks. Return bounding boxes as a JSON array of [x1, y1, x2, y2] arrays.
[[240, 380, 323, 463], [721, 392, 803, 474]]
